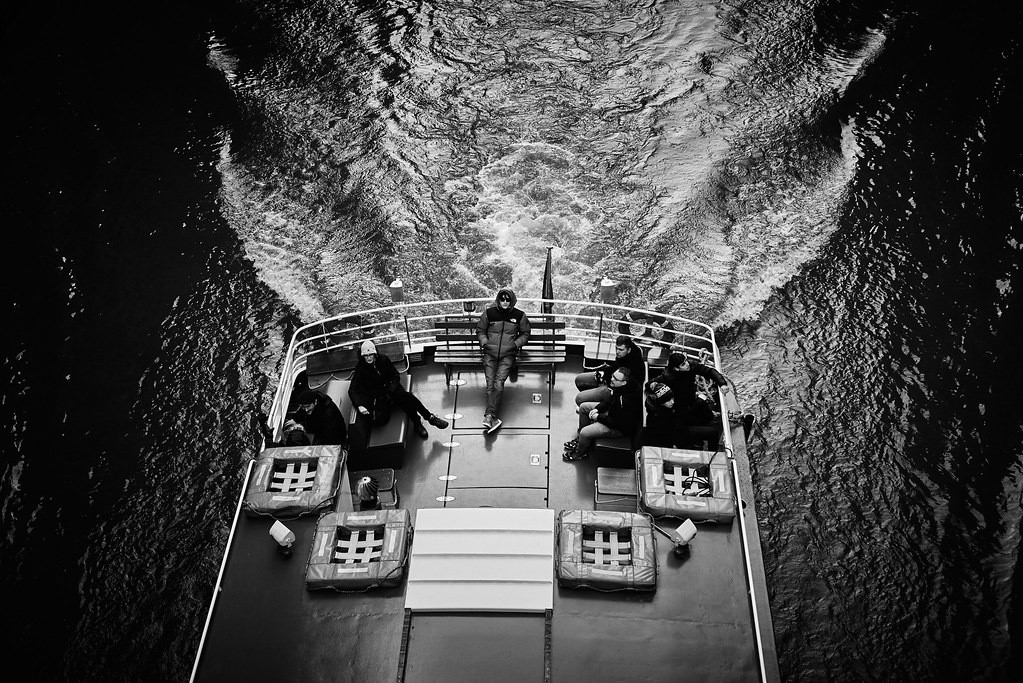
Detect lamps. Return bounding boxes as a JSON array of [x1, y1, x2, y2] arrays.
[[269, 520, 296, 550], [671, 518, 698, 549]]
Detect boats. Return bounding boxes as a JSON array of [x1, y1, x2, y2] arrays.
[[191, 277, 780, 683]]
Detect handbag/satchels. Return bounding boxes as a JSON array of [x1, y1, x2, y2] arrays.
[[362, 382, 392, 426], [511, 360, 518, 382]]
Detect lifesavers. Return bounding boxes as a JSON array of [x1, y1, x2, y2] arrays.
[[618, 311, 676, 349]]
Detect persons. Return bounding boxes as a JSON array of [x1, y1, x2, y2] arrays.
[[476, 289, 531, 434], [348, 341, 449, 439], [283, 391, 346, 448], [562, 336, 644, 460], [645, 354, 729, 450]]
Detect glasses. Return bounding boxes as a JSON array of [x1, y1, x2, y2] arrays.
[[612, 373, 627, 382], [363, 354, 374, 358], [500, 297, 510, 302]]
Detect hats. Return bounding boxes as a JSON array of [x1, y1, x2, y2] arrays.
[[360, 340, 377, 356], [649, 382, 674, 404]]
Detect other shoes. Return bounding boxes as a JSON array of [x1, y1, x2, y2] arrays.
[[414, 423, 428, 439], [429, 414, 449, 429]]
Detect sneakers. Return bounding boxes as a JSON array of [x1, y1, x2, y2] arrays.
[[483, 415, 493, 429], [562, 448, 591, 461], [564, 438, 580, 449], [488, 418, 503, 434]]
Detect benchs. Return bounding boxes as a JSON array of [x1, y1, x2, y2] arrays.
[[367, 374, 411, 461], [434, 322, 566, 384], [306, 341, 405, 389], [595, 467, 637, 507], [350, 469, 399, 508], [583, 342, 670, 370], [594, 362, 648, 451], [323, 379, 351, 433]]
